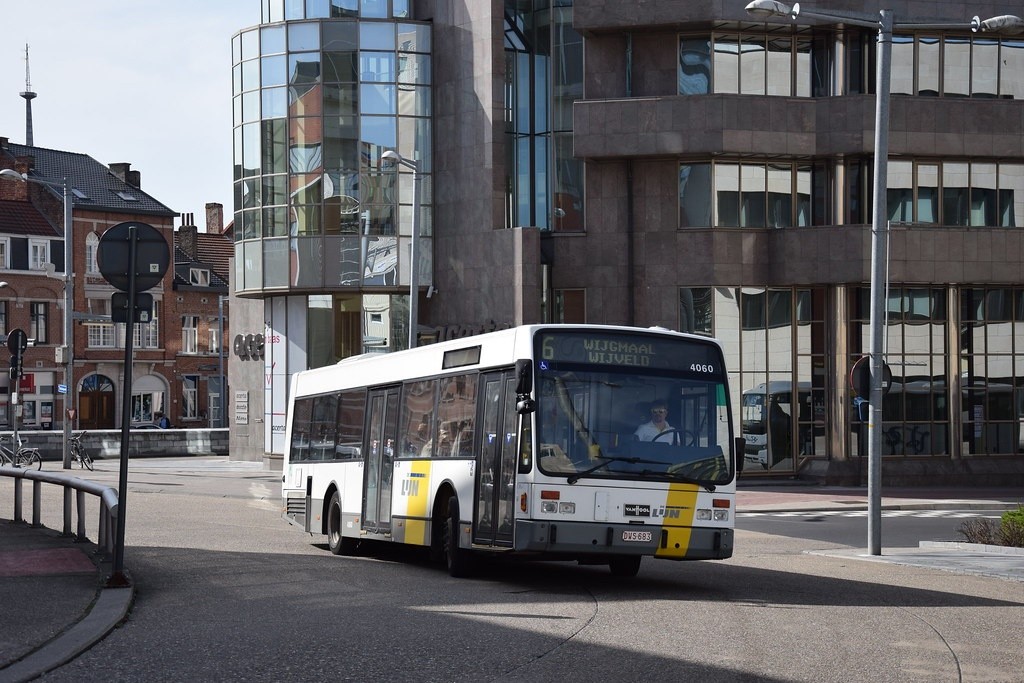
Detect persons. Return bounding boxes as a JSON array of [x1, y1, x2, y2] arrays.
[[632, 399, 681, 446], [157, 413, 171, 429], [410, 420, 471, 457]]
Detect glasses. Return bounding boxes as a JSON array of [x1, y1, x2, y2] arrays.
[[651, 409, 667, 414]]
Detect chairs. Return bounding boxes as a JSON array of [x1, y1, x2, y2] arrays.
[[293, 441, 363, 460]]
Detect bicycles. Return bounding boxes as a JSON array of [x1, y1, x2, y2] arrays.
[[67, 430, 95, 472], [0, 435, 42, 471]]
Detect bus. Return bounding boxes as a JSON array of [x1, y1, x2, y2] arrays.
[[734, 379, 1019, 471], [281, 324, 747, 580]]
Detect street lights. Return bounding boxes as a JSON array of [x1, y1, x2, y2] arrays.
[[382, 149, 424, 349], [742, 1, 1024, 557], [0, 169, 75, 540]]
